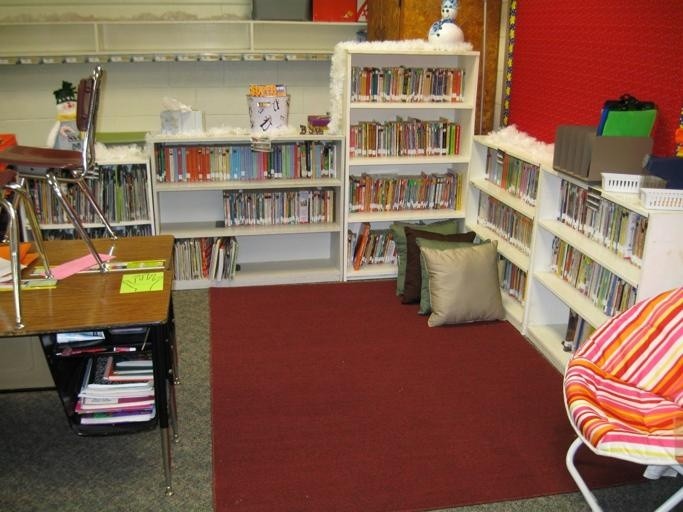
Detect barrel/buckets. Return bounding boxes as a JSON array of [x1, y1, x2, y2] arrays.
[[249, 94, 290, 130]]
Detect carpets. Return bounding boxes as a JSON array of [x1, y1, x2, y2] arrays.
[[208, 281, 650, 512]]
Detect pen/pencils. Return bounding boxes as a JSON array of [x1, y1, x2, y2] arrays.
[[56, 348, 107, 355]]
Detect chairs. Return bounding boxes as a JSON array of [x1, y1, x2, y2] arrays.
[[1, 66, 117, 273], [562, 287, 683, 512], [0, 168, 53, 330]]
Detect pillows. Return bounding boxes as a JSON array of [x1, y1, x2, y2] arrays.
[[391, 219, 506, 328]]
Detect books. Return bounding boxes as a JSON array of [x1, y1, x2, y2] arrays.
[[154, 144, 337, 183], [24, 165, 152, 240], [55, 327, 156, 425], [223, 189, 334, 227], [347, 64, 464, 270], [175, 237, 239, 280], [474, 148, 648, 354]]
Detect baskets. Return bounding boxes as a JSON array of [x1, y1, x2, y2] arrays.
[[600, 172, 667, 193], [640, 187, 683, 211]]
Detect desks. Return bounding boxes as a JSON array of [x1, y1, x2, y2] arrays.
[[0, 271, 180, 497], [0, 234, 181, 387]]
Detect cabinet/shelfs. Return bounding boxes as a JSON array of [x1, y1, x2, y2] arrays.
[[149, 134, 345, 292], [21, 159, 156, 242], [463, 134, 553, 335], [0, 21, 366, 66], [343, 46, 481, 283], [524, 164, 683, 377]]
